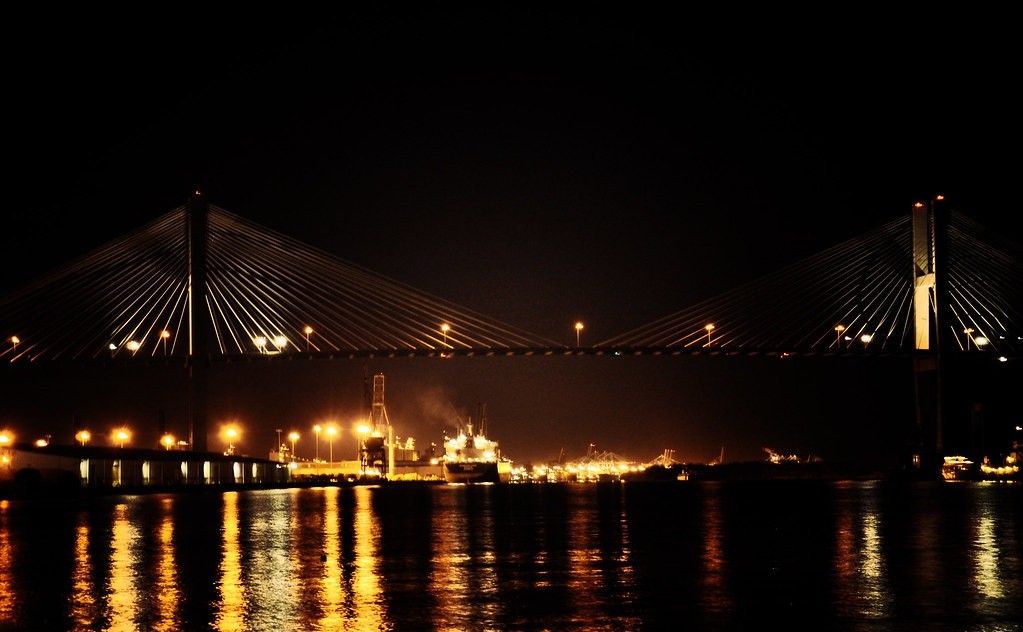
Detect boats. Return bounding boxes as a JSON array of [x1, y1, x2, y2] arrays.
[[442, 416, 511, 486], [941, 441, 1023, 484]]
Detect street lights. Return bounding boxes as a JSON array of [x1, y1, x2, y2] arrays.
[[290, 432, 298, 461], [305, 326, 312, 350], [705, 323, 715, 347], [163, 437, 172, 451], [226, 429, 237, 455], [160, 331, 170, 355], [964, 327, 974, 350], [575, 322, 584, 346], [914, 195, 944, 348], [80, 433, 87, 446], [118, 430, 127, 448], [12, 336, 18, 355], [327, 427, 336, 468], [313, 425, 321, 459], [441, 323, 449, 349], [837, 325, 844, 347]]
